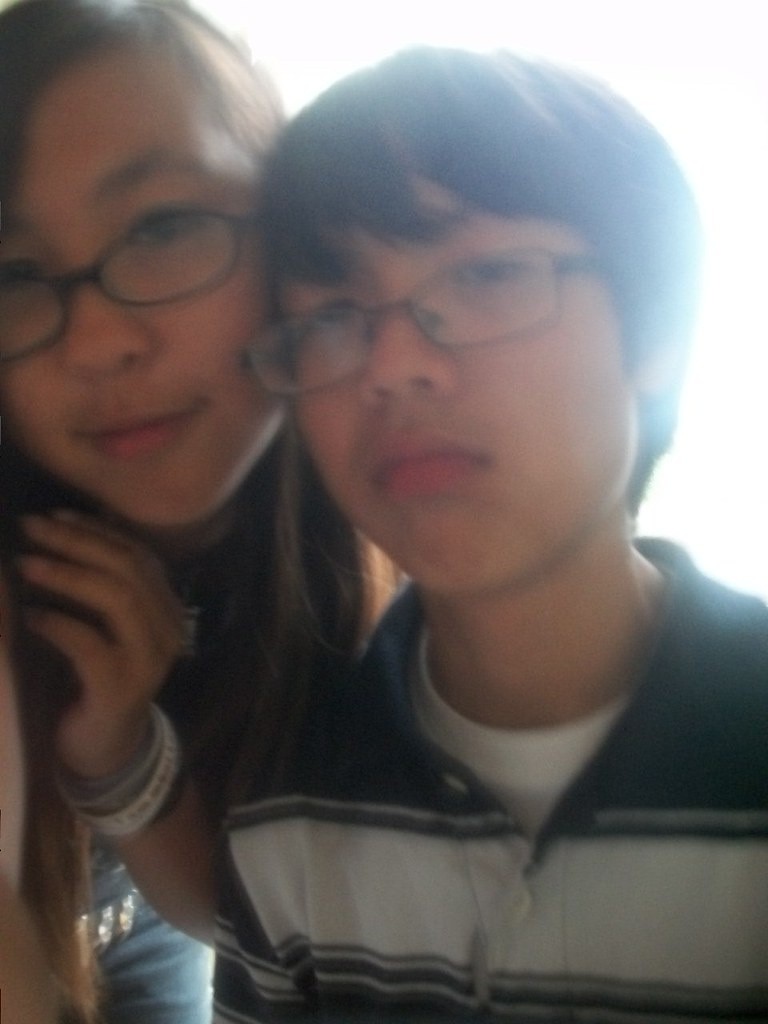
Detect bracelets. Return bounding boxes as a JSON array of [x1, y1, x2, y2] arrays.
[[59, 701, 186, 847]]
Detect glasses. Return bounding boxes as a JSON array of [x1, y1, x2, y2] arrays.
[[238, 245, 607, 400], [0, 206, 268, 362]]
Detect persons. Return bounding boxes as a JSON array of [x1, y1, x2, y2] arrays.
[[212, 47, 768, 1024], [0, 0, 395, 1024]]
